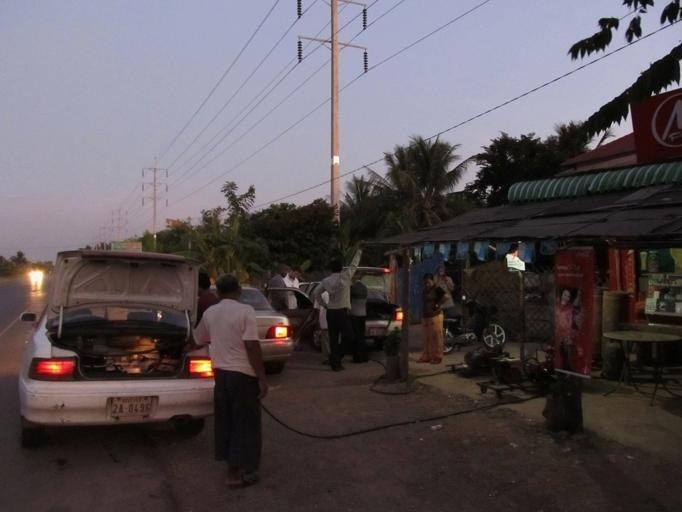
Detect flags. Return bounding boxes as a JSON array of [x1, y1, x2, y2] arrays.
[[413, 238, 576, 265]]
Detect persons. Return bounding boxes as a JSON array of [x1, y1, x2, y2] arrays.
[[281, 265, 302, 311], [347, 269, 369, 363], [433, 265, 455, 335], [263, 262, 289, 312], [311, 290, 341, 365], [196, 271, 219, 327], [554, 288, 574, 371], [183, 274, 268, 489], [418, 273, 449, 364], [312, 240, 367, 371]]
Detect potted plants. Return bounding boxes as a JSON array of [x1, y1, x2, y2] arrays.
[[382, 327, 400, 380]]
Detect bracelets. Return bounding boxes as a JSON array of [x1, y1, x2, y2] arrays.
[[435, 304, 440, 308]]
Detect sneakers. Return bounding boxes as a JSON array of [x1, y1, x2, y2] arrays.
[[332, 344, 369, 371]]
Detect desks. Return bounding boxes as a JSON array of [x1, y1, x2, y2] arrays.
[[602, 331, 682, 407]]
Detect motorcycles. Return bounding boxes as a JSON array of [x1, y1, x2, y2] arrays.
[[443, 289, 507, 354]]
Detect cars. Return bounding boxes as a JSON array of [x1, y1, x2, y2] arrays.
[[298, 268, 404, 347], [208, 284, 314, 374], [19, 251, 215, 442]]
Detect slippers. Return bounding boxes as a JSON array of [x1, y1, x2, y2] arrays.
[[416, 356, 441, 364], [224, 475, 256, 490]]
